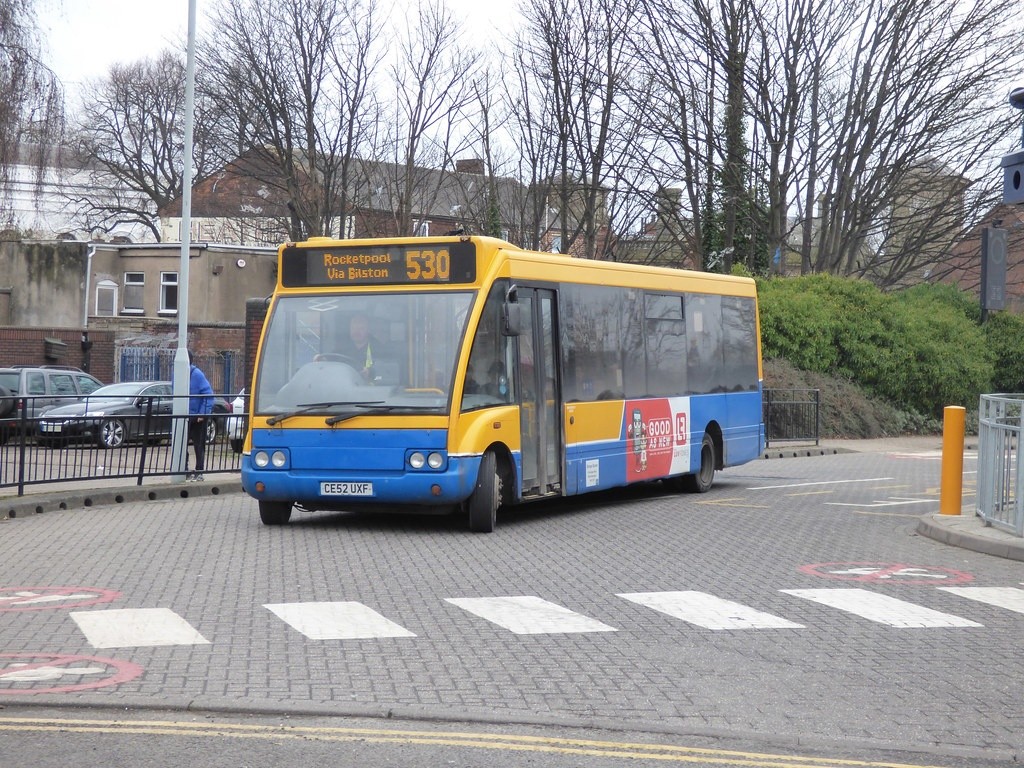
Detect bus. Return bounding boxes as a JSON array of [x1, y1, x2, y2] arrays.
[[239, 234, 765, 534]]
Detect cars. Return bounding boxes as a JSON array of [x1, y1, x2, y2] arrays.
[[34, 380, 232, 448], [226, 386, 245, 455]]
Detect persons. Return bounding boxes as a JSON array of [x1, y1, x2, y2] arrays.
[[331, 312, 382, 381], [173, 350, 215, 481]]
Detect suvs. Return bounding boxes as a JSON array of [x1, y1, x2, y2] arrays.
[[0, 364, 107, 445]]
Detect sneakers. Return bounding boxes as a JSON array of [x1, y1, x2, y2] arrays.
[[186, 474, 193, 482], [190, 473, 205, 482]]
